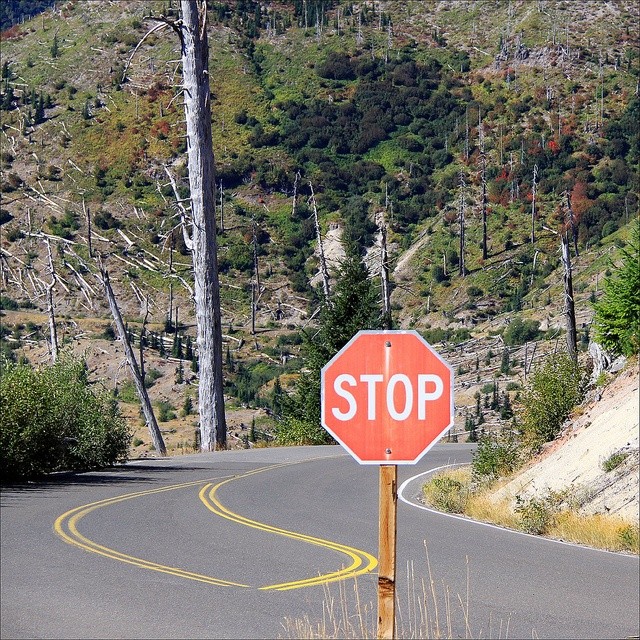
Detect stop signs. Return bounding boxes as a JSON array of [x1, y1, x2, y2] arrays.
[[321, 330, 454, 463]]
